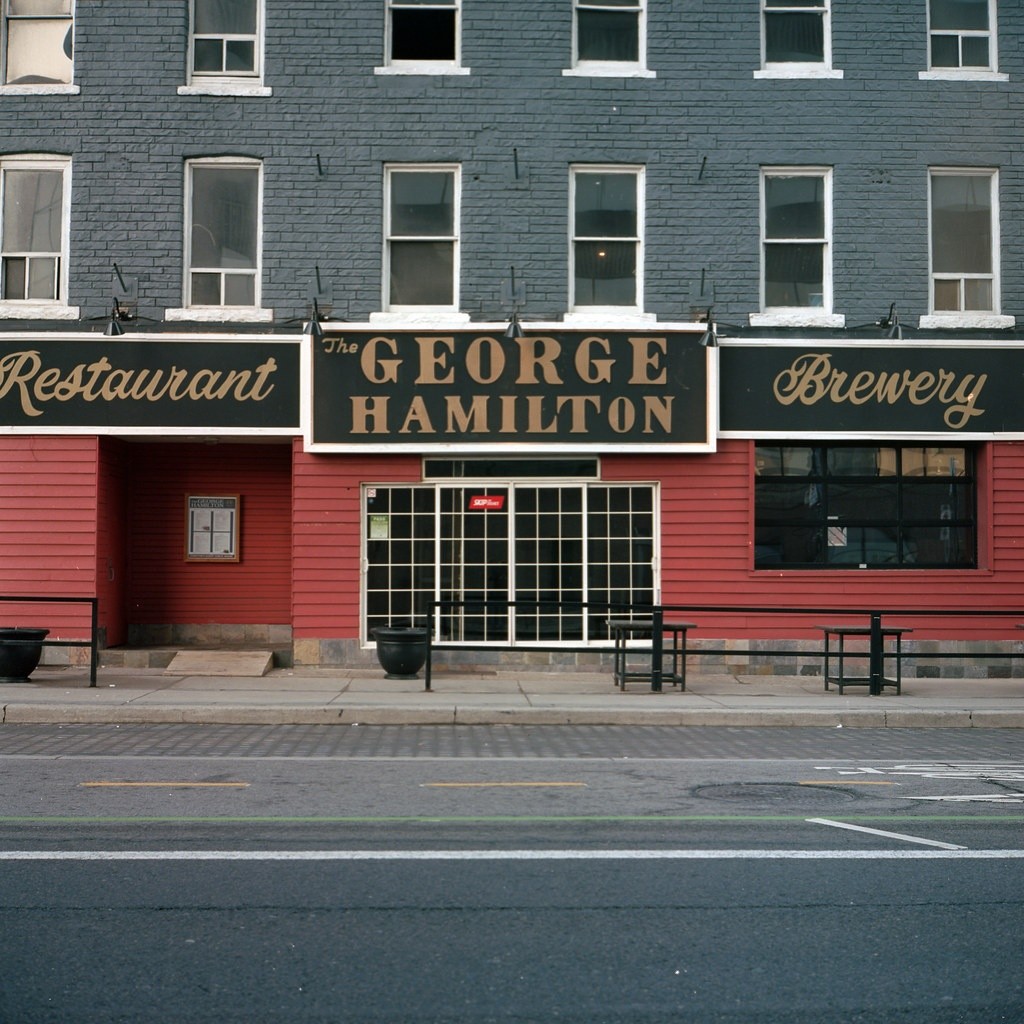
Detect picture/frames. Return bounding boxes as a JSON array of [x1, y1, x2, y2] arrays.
[[183, 493, 240, 562]]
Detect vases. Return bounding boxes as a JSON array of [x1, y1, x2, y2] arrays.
[[0, 626, 50, 683], [369, 627, 436, 679]]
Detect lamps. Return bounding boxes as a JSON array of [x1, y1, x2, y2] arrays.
[[485, 300, 546, 338], [84, 297, 160, 337], [844, 302, 919, 340], [282, 297, 349, 336], [698, 320, 743, 347]]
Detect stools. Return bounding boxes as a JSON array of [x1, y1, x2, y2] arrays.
[[815, 623, 913, 695], [605, 620, 698, 692]]
